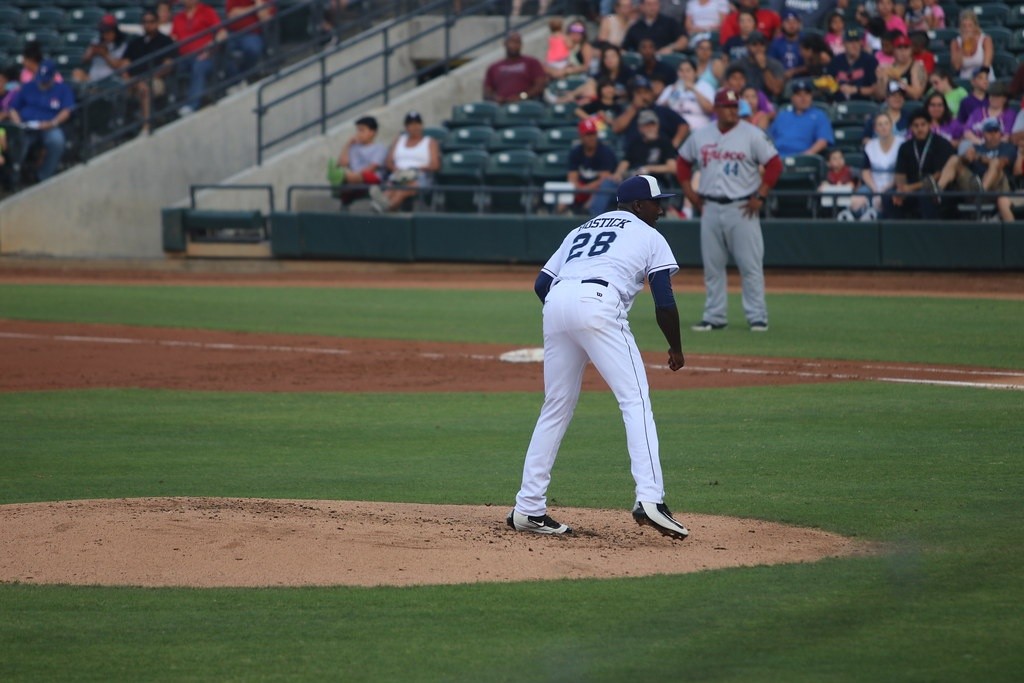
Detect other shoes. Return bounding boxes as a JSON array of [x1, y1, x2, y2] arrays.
[[367, 186, 388, 214], [750, 322, 768, 331], [691, 321, 726, 331]]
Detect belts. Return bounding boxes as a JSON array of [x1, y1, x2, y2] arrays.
[[554, 279, 608, 287], [706, 195, 749, 204]]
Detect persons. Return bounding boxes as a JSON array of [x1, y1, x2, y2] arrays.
[[332, 112, 441, 211], [482, 1, 1024, 222], [505, 174, 690, 538], [676, 86, 783, 332], [1, 0, 276, 192]]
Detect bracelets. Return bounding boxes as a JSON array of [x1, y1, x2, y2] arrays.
[[754, 192, 765, 201], [519, 92, 528, 100]]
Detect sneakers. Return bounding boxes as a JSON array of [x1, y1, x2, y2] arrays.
[[506, 508, 572, 534], [632, 502, 689, 541]]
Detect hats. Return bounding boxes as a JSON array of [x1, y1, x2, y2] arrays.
[[887, 82, 904, 95], [638, 111, 657, 125], [983, 122, 1001, 132], [405, 111, 420, 123], [579, 119, 597, 134], [972, 66, 989, 78], [845, 29, 862, 41], [35, 61, 55, 84], [714, 88, 740, 106], [792, 81, 810, 93], [894, 37, 911, 46], [616, 174, 677, 202]]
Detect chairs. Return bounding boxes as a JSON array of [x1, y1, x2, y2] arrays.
[[0, 0, 1024, 220]]
